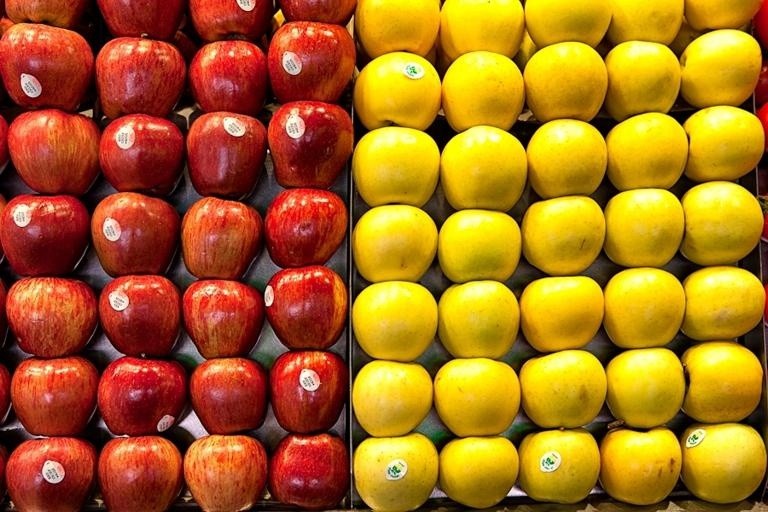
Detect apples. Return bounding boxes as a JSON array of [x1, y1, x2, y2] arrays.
[[352, 360, 434, 436], [442, 50, 525, 131], [681, 341, 764, 423], [0, 194, 90, 277], [678, 180, 764, 266], [603, 187, 685, 269], [98, 356, 189, 436], [279, 0, 357, 26], [189, 0, 268, 40], [8, 354, 99, 438], [356, 0, 441, 57], [4, 437, 101, 512], [182, 434, 267, 512], [604, 267, 686, 348], [7, 107, 100, 193], [268, 100, 353, 189], [438, 209, 523, 282], [264, 187, 348, 268], [527, 117, 608, 199], [181, 196, 264, 280], [522, 194, 606, 275], [681, 423, 767, 505], [600, 425, 681, 504], [4, 277, 96, 355], [91, 193, 180, 276], [680, 266, 766, 341], [438, 436, 519, 510], [270, 349, 346, 432], [523, 40, 608, 121], [189, 356, 269, 435], [440, 124, 528, 210], [685, 1, 764, 29], [434, 357, 521, 437], [442, 0, 525, 60], [0, 23, 96, 108], [189, 39, 268, 111], [269, 20, 357, 104], [351, 204, 438, 281], [265, 265, 345, 349], [605, 112, 688, 192], [682, 106, 766, 181], [525, 0, 612, 50], [99, 436, 182, 511], [520, 275, 605, 353], [96, 37, 187, 117], [352, 280, 438, 362], [518, 428, 601, 504], [438, 280, 521, 358], [604, 347, 686, 429], [267, 432, 350, 508], [354, 432, 438, 511], [353, 126, 440, 208], [97, 274, 181, 356], [101, 114, 184, 192], [96, 1, 181, 36], [186, 111, 268, 197], [353, 51, 442, 130], [3, 0, 80, 26], [608, 0, 685, 44], [519, 349, 607, 428], [181, 279, 264, 360]]
